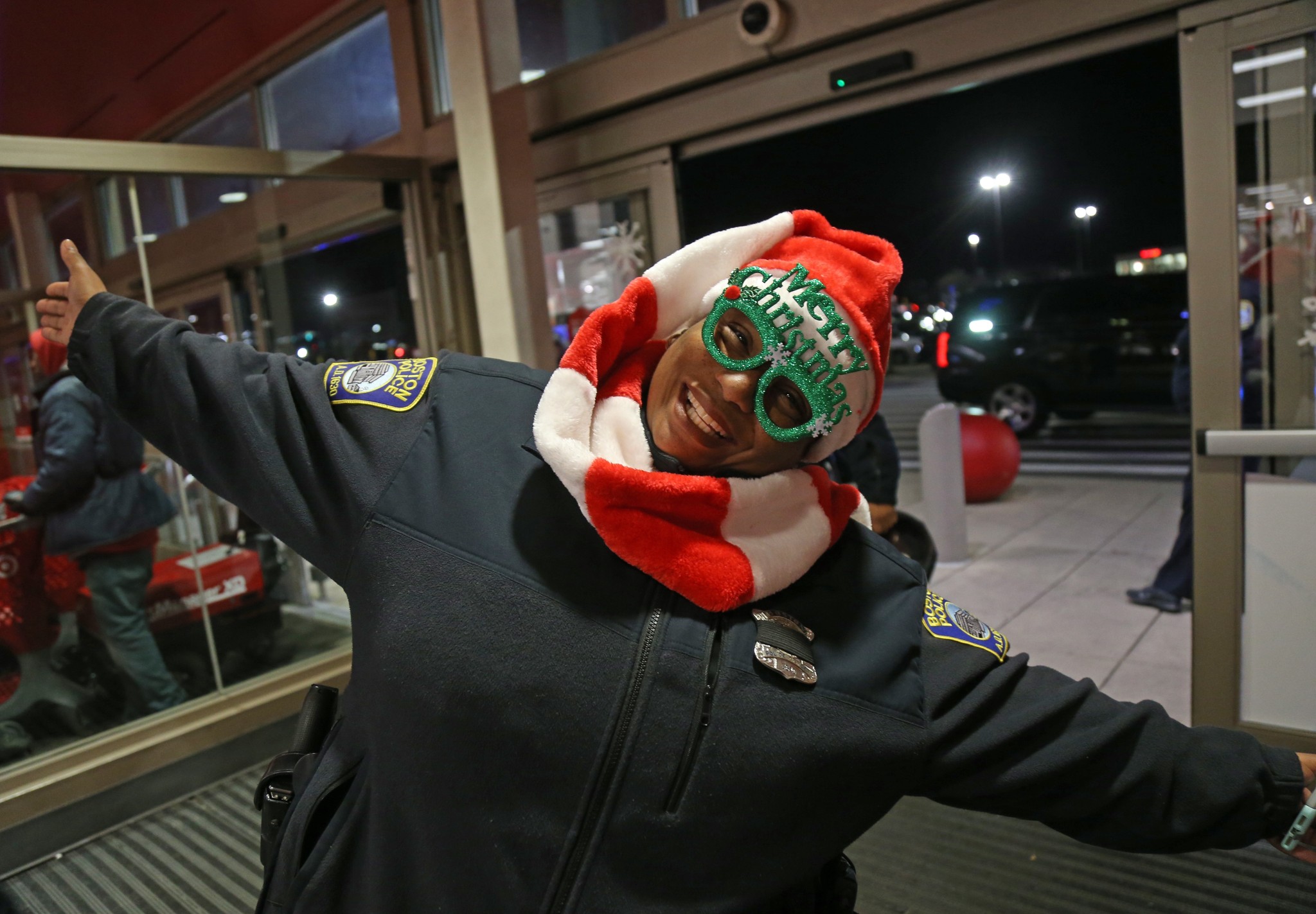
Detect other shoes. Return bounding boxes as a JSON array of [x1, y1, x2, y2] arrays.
[[1125, 585, 1181, 613]]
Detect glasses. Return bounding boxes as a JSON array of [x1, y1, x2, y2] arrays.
[[702, 287, 832, 442]]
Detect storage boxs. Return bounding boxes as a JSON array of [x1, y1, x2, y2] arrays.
[[76, 543, 266, 641]]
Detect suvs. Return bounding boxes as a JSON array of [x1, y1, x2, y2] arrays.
[[938, 271, 1184, 442]]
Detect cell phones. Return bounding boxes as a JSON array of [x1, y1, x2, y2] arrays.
[[1279, 783, 1316, 851]]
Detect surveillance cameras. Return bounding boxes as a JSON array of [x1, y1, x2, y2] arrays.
[[734, 0, 789, 50]]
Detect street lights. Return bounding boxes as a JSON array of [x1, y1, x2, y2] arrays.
[[978, 173, 1011, 261], [1077, 205, 1096, 251]]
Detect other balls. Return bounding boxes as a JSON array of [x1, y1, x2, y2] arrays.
[[959, 406, 1021, 500]]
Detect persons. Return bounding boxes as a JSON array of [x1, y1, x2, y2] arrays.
[[1124, 278, 1246, 615], [815, 410, 898, 535], [3, 326, 187, 724], [34, 208, 1316, 914]]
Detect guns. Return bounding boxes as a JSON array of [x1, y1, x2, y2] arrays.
[[254, 683, 339, 865]]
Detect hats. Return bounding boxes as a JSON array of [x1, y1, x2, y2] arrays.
[[533, 207, 903, 612]]
